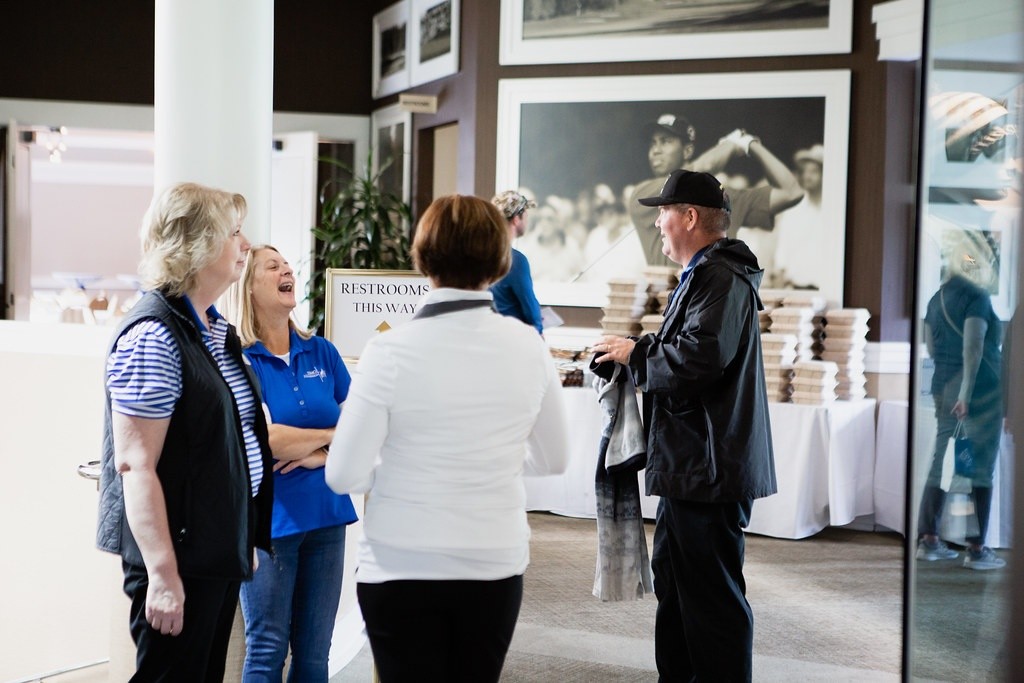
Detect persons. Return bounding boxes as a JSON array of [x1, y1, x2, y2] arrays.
[[95, 183, 275, 683], [516, 115, 827, 305], [217, 242, 360, 683], [323, 195, 574, 683], [914, 230, 1006, 570], [490, 190, 545, 340], [586, 169, 779, 683], [928, 89, 1021, 295]]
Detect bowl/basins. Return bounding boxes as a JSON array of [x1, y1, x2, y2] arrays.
[[598, 265, 870, 404]]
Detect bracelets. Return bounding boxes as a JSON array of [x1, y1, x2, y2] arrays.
[[323, 446, 329, 454]]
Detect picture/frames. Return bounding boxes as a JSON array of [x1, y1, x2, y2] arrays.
[[372, 0, 460, 99], [493, 69, 852, 308], [498, 0, 853, 64]]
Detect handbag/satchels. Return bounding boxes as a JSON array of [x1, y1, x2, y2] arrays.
[[939, 418, 974, 493]]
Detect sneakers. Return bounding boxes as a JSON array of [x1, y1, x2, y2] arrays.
[[915, 539, 958, 562], [963, 547, 1007, 570]]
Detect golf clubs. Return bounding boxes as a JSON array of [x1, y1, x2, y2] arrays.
[[563, 227, 635, 283]]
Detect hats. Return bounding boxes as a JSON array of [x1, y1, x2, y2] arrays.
[[638, 169, 730, 211], [644, 113, 695, 143], [794, 144, 823, 166], [491, 190, 536, 218], [933, 93, 1008, 151]]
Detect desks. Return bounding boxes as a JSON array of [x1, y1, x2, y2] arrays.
[[874, 400, 1015, 550], [523, 358, 875, 540]]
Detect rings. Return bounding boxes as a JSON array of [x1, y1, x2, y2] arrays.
[[605, 344, 611, 352]]
